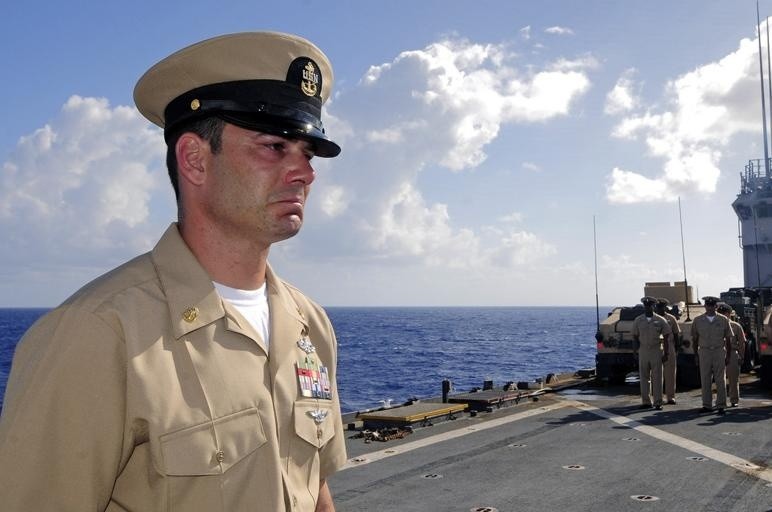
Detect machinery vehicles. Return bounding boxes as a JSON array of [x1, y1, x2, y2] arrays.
[[590, 195, 771, 387]]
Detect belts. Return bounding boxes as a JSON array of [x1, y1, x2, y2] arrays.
[[640, 344, 660, 349], [698, 345, 724, 350]]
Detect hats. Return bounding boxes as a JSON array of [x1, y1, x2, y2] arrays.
[[702, 296, 721, 305], [133, 31, 341, 158], [717, 303, 732, 314], [641, 296, 656, 305], [655, 298, 670, 306]]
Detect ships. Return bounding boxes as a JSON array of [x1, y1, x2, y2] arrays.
[[322, 1, 770, 510]]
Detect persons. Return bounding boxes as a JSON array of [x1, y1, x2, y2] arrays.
[[0, 32, 347, 512], [633, 297, 673, 410], [655, 297, 681, 403], [690, 296, 734, 412], [716, 302, 748, 407]]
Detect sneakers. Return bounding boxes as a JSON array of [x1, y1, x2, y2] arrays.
[[655, 404, 663, 410], [642, 402, 652, 408], [667, 398, 676, 405], [652, 400, 664, 406], [718, 408, 725, 415], [700, 406, 712, 412], [731, 402, 738, 407], [712, 402, 727, 409]]
